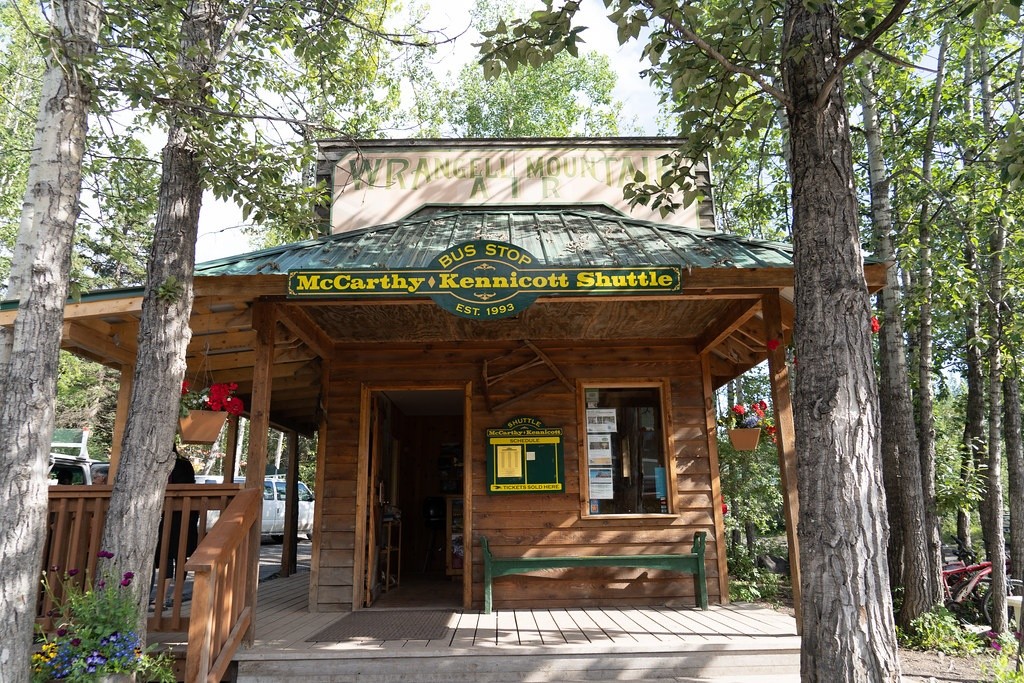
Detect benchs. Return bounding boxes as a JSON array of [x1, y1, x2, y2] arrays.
[[479, 531, 709, 611]]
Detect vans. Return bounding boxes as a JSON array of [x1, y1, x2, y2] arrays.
[[188, 475, 315, 542]]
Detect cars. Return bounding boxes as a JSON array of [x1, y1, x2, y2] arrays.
[[44, 452, 109, 486]]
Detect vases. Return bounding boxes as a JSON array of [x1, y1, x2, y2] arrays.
[[99, 671, 136, 683], [728, 427, 761, 450], [176, 409, 229, 445]]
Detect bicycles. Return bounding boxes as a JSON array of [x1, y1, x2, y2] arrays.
[[927, 534, 1024, 633]]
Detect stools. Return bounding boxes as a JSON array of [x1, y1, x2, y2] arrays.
[[420, 497, 447, 576]]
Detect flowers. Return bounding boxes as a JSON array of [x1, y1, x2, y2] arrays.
[[178, 379, 244, 420], [30, 550, 177, 683], [717, 400, 777, 444]]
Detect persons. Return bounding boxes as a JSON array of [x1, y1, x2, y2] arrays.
[[148, 442, 199, 610], [71, 466, 111, 570]]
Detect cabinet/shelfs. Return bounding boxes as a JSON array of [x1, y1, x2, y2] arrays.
[[380, 517, 402, 593], [445, 494, 464, 580]]
